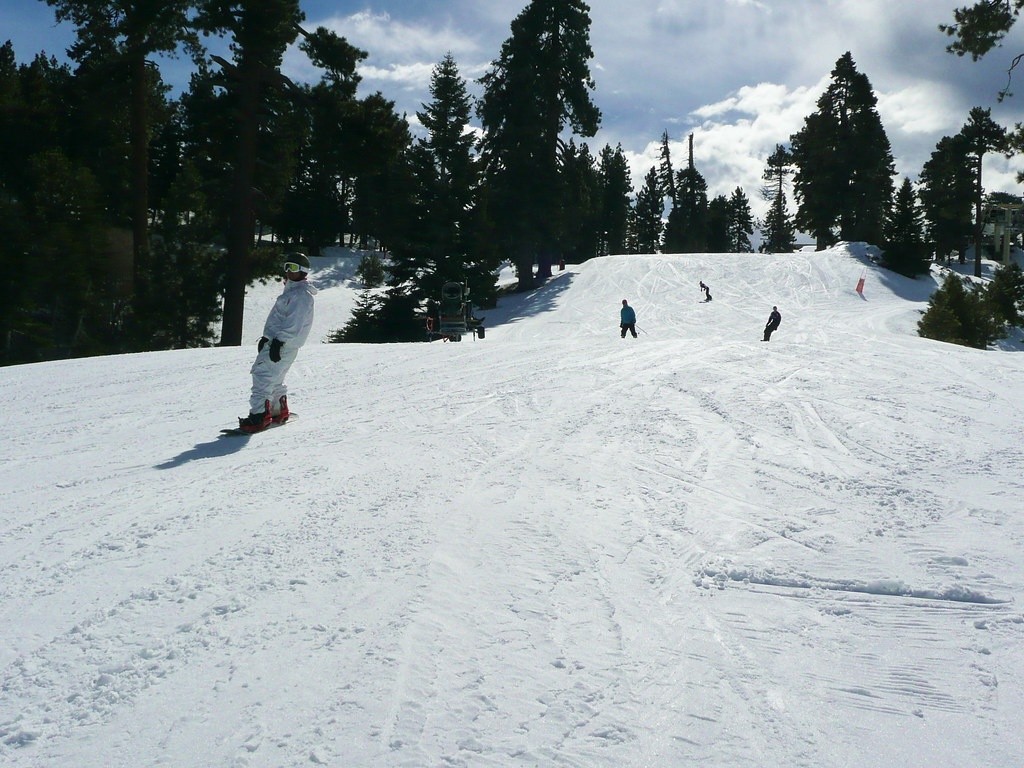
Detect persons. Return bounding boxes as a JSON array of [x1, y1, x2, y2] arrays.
[[699, 281, 712, 301], [239, 252, 318, 435], [764, 306, 781, 341], [620, 300, 637, 339]]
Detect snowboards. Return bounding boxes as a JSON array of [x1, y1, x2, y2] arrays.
[[219, 412, 298, 436]]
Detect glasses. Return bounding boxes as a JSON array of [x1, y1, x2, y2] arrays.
[[284, 262, 300, 273]]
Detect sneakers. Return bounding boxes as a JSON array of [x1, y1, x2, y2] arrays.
[[271, 410, 288, 422], [239, 411, 270, 432]]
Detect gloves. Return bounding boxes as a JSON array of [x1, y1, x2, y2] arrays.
[[268, 336, 285, 363], [255, 336, 269, 354]]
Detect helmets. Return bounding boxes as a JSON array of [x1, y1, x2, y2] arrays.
[[286, 252, 310, 276]]
